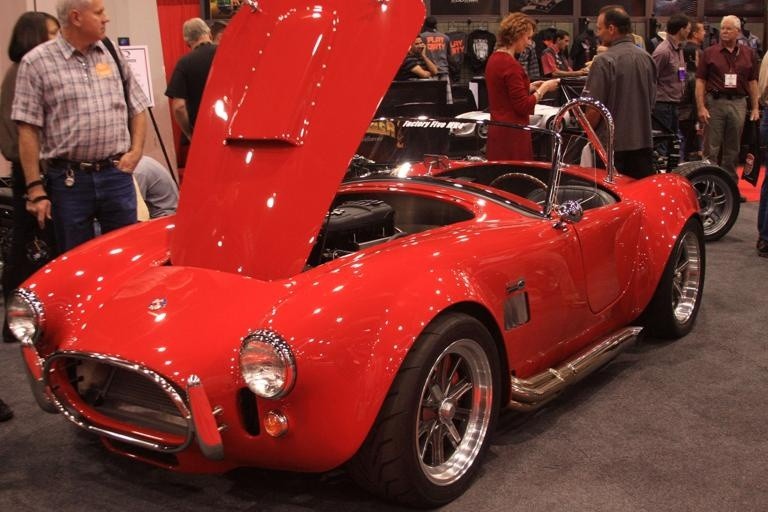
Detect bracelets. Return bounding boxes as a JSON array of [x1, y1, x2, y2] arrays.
[[536, 90, 543, 99]]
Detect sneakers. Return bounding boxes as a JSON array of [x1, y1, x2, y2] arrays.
[[0, 399, 14, 423], [755, 236, 768, 258]]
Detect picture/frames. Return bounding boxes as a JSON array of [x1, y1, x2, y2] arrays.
[[119, 45, 155, 108]]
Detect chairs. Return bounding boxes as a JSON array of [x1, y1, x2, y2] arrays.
[[525, 185, 616, 211]]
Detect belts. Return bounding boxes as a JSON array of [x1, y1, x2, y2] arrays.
[[707, 92, 748, 100], [45, 152, 124, 175]]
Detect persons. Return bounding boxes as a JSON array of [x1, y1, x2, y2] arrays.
[[1, 1, 178, 342], [165, 17, 226, 143], [484, 12, 561, 160], [756, 53, 768, 254], [583, 5, 655, 179], [653, 14, 759, 203], [395, 34, 438, 79], [516, 31, 586, 80]]
